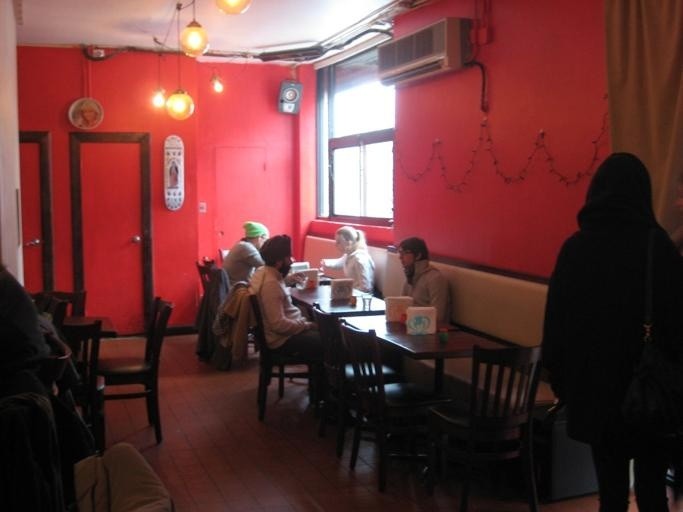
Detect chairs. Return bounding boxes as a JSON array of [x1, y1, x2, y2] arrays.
[[429, 344, 542, 512], [29, 288, 86, 318], [94, 297, 174, 455]]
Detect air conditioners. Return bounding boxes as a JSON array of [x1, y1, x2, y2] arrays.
[[378, 16, 478, 90]]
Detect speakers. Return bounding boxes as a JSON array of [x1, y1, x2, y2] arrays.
[[277, 81, 303, 113]]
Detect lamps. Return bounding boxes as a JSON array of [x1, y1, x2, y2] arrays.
[[166, 3, 194, 120], [210, 73, 224, 94], [152, 55, 166, 108], [179, 1, 209, 57], [215, 0, 253, 17]]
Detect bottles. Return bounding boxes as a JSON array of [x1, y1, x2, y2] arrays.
[[440, 328, 448, 343]]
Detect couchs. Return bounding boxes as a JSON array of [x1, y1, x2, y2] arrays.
[[304, 234, 558, 417]]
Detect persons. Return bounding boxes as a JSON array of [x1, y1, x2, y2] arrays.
[[397, 237, 453, 322], [0, 259, 87, 413], [250, 234, 328, 420], [321, 225, 378, 294], [541, 153, 683, 512], [221, 221, 270, 288]]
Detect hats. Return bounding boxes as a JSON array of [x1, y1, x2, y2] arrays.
[[245, 222, 268, 238]]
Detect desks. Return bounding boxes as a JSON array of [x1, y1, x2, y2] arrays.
[[53, 316, 118, 361]]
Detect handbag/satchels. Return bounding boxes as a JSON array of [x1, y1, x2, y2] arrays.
[[622, 326, 683, 449]]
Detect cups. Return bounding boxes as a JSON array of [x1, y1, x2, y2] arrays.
[[296, 275, 308, 289], [362, 293, 372, 311]]
